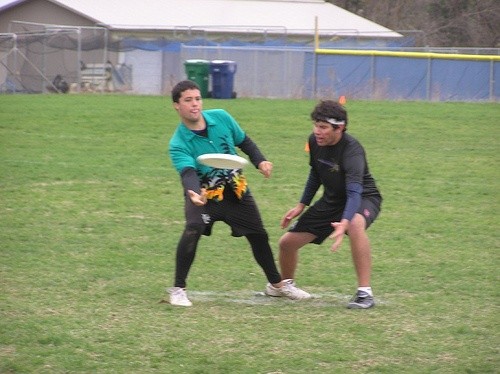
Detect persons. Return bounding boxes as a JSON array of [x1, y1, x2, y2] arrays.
[[279, 102, 383, 308], [167, 81, 312, 307]]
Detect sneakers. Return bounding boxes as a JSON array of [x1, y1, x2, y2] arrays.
[[347, 290, 375, 309], [266, 281, 312, 300], [167, 287, 192, 307]]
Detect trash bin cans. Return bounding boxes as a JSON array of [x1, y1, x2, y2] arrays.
[[184, 59, 209, 98], [210, 60, 237, 98]]
